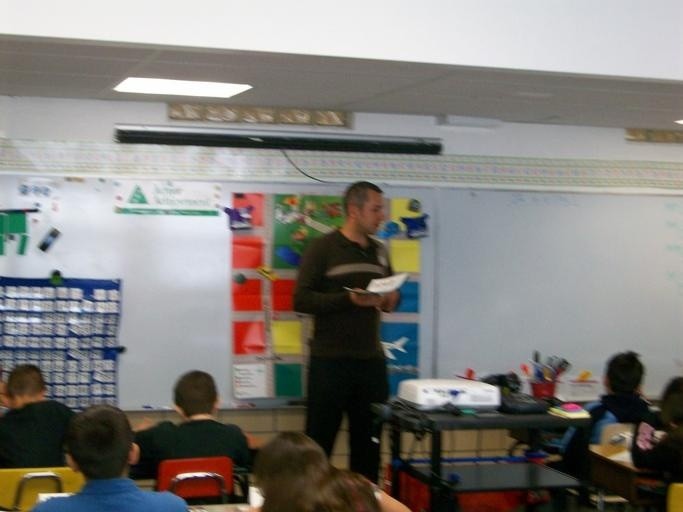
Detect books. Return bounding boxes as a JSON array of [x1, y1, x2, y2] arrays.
[[342, 271, 412, 299], [548, 402, 591, 420], [236, 485, 266, 512], [35, 491, 74, 505], [648, 428, 668, 445]]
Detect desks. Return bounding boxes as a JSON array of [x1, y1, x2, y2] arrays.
[[373, 396, 673, 512], [188, 503, 265, 512], [128, 471, 263, 492]]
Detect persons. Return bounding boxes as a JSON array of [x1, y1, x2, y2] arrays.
[[28, 404, 189, 512], [252, 426, 414, 512], [630, 373, 682, 512], [129, 370, 261, 480], [289, 177, 403, 489], [0, 363, 77, 469], [534, 348, 650, 511]]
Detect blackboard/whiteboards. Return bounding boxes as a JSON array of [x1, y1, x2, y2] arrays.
[[0, 170, 683, 415]]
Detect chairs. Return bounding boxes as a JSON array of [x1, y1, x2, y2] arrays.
[[157, 456, 234, 506], [0, 467, 86, 512], [667, 483, 683, 512]]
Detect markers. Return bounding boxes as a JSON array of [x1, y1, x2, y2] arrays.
[[466, 366, 474, 379]]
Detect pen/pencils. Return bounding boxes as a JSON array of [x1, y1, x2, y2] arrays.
[[519, 351, 570, 382]]
